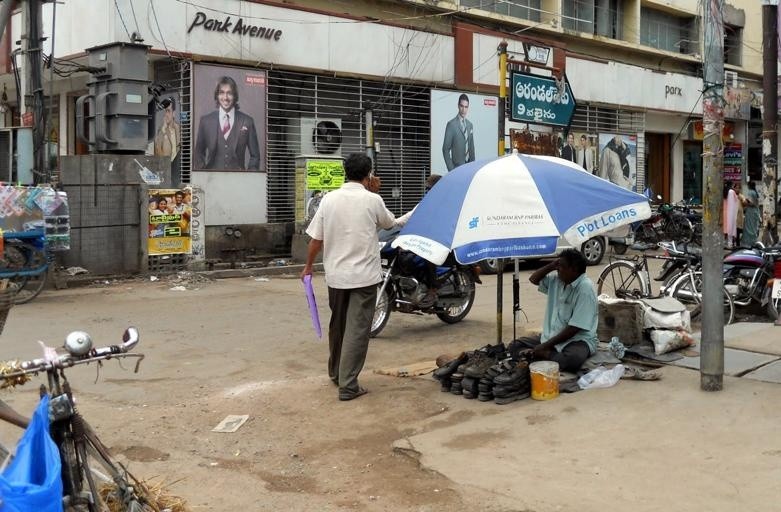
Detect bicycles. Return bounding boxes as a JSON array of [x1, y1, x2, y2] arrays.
[[597, 195, 781, 325], [0, 326, 144, 512]]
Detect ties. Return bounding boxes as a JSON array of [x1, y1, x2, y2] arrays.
[[618, 145, 629, 178], [582, 150, 587, 171], [223, 114, 232, 141], [461, 119, 470, 163]]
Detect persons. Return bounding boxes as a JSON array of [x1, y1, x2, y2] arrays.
[[508, 247, 601, 371], [364, 169, 383, 196], [563, 133, 577, 167], [723, 180, 744, 251], [601, 136, 638, 192], [743, 182, 763, 249], [157, 97, 181, 161], [442, 93, 476, 175], [171, 190, 190, 215], [150, 198, 168, 216], [301, 152, 399, 402], [387, 173, 456, 307], [577, 134, 595, 174], [195, 75, 260, 171], [732, 184, 747, 249]]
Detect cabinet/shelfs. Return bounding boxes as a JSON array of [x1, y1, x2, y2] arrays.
[[724, 145, 745, 195]]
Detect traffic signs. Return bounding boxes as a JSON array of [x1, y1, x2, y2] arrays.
[[508, 70, 576, 137]]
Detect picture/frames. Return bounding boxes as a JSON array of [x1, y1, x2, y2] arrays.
[[191, 61, 267, 172], [555, 130, 598, 177], [597, 131, 637, 192], [429, 88, 499, 177]]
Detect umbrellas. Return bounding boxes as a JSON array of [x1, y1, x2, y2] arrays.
[[390, 148, 654, 341]]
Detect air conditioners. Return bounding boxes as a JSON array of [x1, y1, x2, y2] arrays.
[[724, 71, 738, 90], [287, 118, 342, 156]]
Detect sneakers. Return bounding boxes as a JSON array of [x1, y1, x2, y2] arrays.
[[432, 341, 531, 405], [338, 383, 369, 402]]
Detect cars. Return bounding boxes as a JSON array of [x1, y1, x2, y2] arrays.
[[479, 236, 605, 274]]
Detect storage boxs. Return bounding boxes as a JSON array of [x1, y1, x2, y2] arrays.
[[597, 303, 645, 345]]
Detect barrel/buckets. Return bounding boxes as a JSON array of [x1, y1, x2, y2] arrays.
[[527, 361, 561, 400]]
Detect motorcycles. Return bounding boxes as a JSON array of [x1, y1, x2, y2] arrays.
[[369, 237, 482, 338]]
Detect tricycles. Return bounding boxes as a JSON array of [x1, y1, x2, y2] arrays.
[[0, 227, 55, 305]]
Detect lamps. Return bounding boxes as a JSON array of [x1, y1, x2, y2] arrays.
[[2, 82, 8, 101]]
